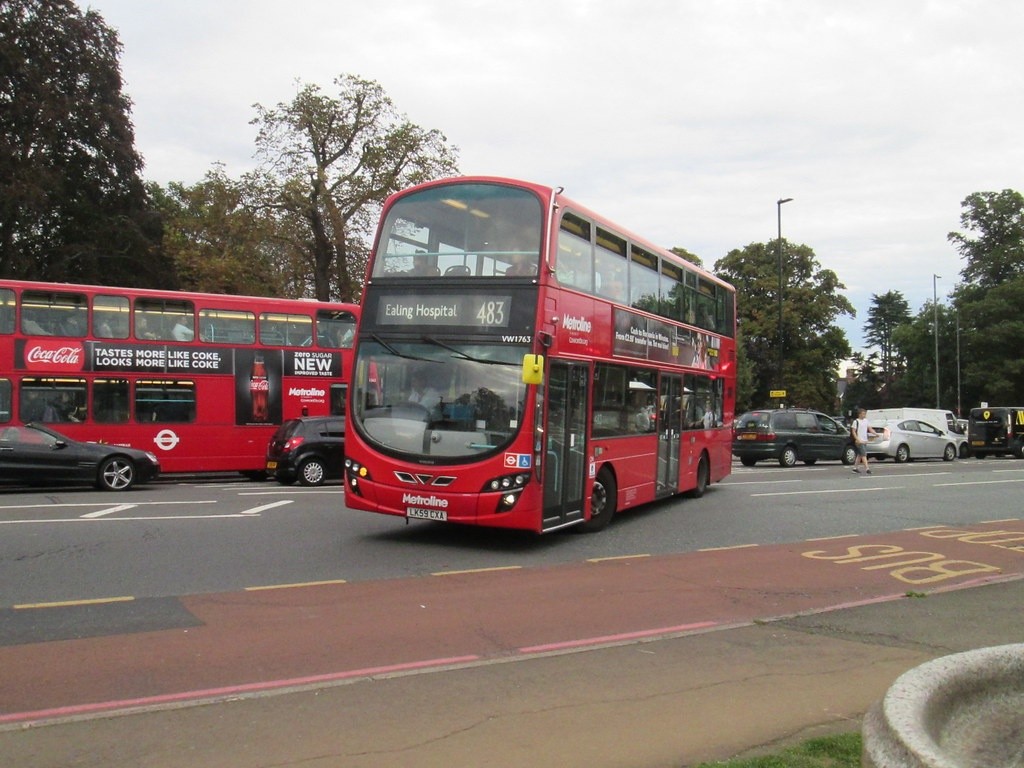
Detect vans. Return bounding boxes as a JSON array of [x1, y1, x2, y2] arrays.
[[968, 407, 1024, 459], [862, 407, 970, 460]]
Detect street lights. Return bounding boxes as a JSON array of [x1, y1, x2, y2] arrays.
[[776, 198, 793, 405], [934, 274, 941, 409]]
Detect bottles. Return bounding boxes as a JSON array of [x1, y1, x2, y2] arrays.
[[249, 350, 270, 424]]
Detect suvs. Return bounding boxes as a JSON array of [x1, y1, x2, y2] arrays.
[[731, 407, 857, 468]]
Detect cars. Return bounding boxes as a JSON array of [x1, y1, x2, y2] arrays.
[[865, 419, 958, 463], [266, 416, 345, 487], [0, 423, 160, 492], [833, 417, 845, 424], [636, 405, 660, 432]]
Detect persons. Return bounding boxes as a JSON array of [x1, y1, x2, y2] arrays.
[[689, 400, 718, 429], [697, 303, 716, 330], [172, 315, 205, 342], [137, 319, 160, 340], [505, 248, 538, 276], [691, 333, 713, 370], [406, 370, 441, 415], [61, 315, 81, 337], [407, 250, 440, 277], [851, 408, 879, 474]]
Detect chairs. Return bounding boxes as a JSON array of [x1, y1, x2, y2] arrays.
[[391, 259, 538, 276], [4, 310, 210, 341], [7, 427, 19, 442], [907, 425, 915, 429], [23, 402, 82, 423]]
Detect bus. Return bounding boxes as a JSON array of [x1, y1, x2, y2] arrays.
[[343, 176, 739, 535], [0, 279, 381, 480]]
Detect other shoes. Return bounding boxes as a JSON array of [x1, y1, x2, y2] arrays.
[[851, 469, 861, 474], [866, 469, 874, 474]]
